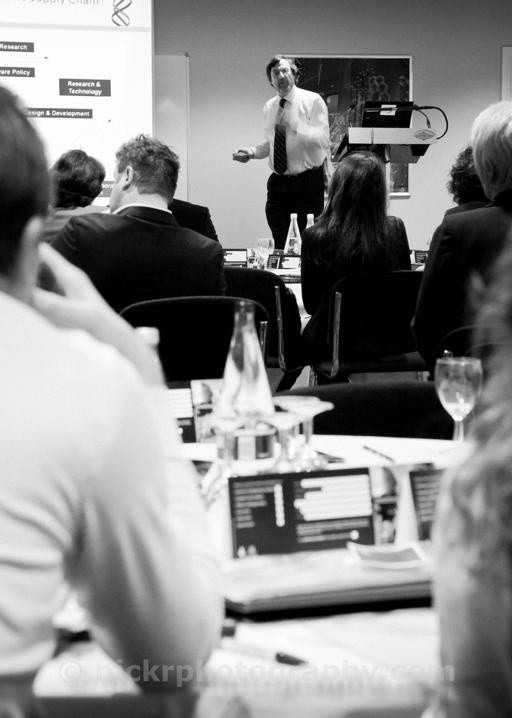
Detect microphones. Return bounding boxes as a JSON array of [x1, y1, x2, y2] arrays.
[[348, 100, 358, 113]]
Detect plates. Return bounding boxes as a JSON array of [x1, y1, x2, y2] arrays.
[[347, 539, 428, 575]]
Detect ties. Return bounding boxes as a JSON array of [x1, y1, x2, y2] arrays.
[[273, 99, 286, 174]]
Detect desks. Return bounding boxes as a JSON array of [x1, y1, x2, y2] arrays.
[[28, 429, 450, 718]]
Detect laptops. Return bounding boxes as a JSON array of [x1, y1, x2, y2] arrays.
[[223, 551, 431, 612]]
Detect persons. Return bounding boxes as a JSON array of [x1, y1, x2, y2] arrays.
[[1, 55, 512, 718]]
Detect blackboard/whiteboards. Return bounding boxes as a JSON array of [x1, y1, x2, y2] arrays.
[[153, 49, 190, 201]]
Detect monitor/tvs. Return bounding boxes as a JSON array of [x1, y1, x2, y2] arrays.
[[362, 100, 413, 127]]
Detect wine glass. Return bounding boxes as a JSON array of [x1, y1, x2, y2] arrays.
[[191, 380, 264, 510], [257, 238, 276, 260], [433, 355, 482, 451], [264, 392, 335, 474]]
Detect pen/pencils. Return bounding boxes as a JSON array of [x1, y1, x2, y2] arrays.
[[216, 636, 305, 665]]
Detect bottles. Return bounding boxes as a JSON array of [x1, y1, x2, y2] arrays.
[[215, 298, 274, 414], [282, 212, 302, 255], [305, 212, 315, 228]]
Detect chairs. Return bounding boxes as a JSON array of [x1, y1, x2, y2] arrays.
[[224, 265, 302, 392], [114, 294, 269, 385], [314, 268, 427, 383]]
